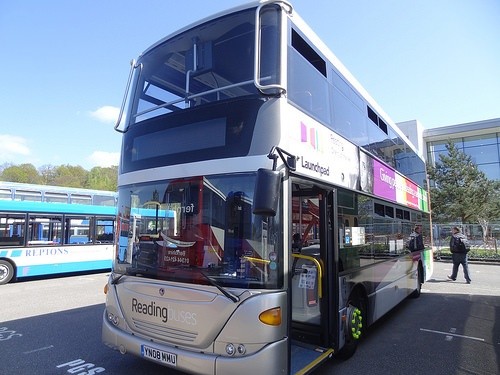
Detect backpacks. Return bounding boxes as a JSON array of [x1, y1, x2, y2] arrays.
[[453, 235, 471, 253]]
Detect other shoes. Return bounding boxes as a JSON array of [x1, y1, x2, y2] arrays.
[[466, 279, 471, 284], [448, 275, 456, 281]]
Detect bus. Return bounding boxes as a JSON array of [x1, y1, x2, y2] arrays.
[[0, 199, 178, 285], [100, 0, 434, 375]]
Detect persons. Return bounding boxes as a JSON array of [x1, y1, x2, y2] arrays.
[[405, 224, 425, 280], [447, 226, 471, 286]]
